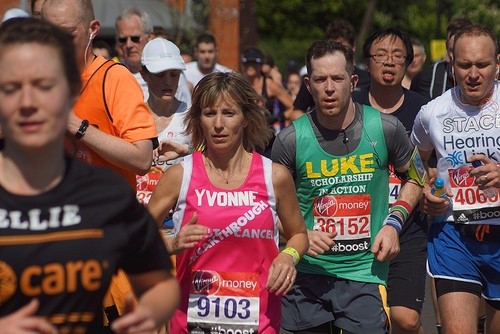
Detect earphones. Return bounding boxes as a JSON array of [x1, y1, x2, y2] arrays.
[[89, 32, 93, 40]]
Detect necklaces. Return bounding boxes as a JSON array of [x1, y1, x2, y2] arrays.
[[205, 155, 247, 184]]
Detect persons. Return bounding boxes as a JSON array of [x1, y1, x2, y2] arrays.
[[0, 0, 500, 334]]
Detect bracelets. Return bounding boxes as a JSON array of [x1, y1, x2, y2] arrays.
[[281, 247, 300, 266], [388, 200, 412, 222], [383, 213, 404, 233], [183, 142, 193, 156], [72, 119, 89, 142]]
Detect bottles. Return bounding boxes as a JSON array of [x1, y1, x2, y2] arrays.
[[430, 178, 446, 222]]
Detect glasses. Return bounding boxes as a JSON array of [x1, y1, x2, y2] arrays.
[[367, 52, 409, 64], [118, 32, 149, 43]]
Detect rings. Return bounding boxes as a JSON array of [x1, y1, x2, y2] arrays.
[[285, 276, 291, 280], [485, 175, 488, 182]]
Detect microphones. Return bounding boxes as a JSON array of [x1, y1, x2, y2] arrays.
[[343, 136, 348, 143]]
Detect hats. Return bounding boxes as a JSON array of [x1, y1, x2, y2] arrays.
[[140, 38, 183, 74], [240, 48, 264, 64]]
